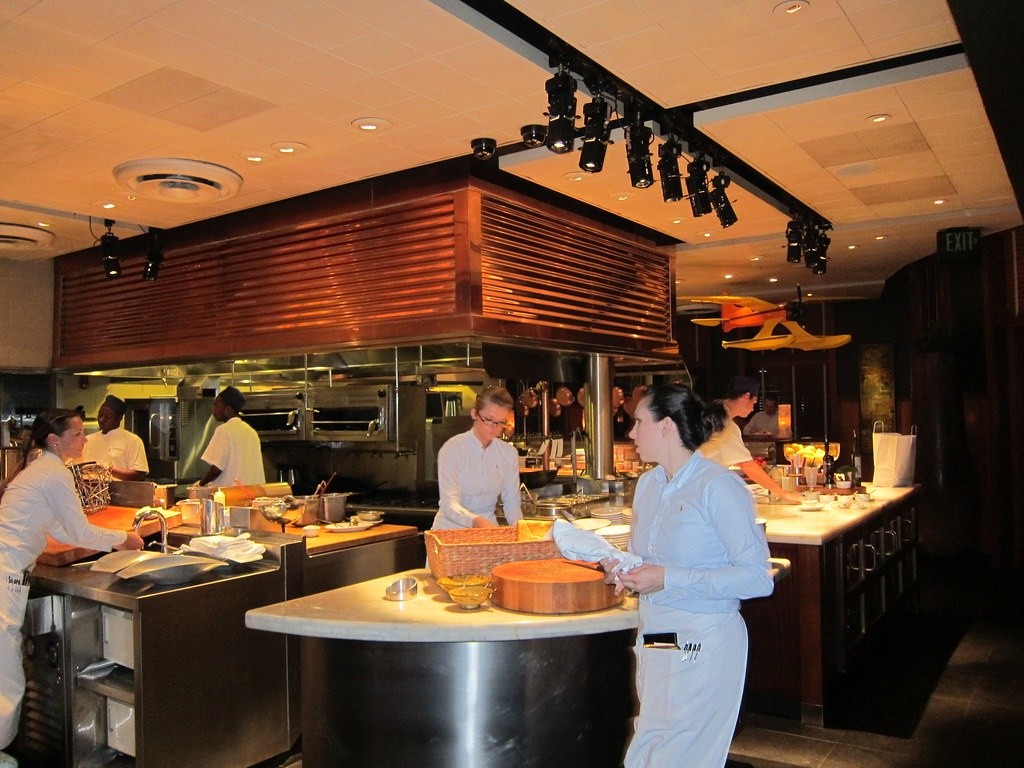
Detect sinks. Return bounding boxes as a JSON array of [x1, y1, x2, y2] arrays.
[[75, 551, 96, 570]]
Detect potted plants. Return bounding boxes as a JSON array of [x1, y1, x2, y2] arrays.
[[837, 465, 858, 483]]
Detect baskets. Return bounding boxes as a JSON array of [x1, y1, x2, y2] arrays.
[[424, 525, 562, 581]]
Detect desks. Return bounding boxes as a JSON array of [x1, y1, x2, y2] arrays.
[[245, 558, 791, 768], [747, 482, 922, 728]]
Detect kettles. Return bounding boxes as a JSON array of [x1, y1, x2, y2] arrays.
[[445, 395, 463, 417]]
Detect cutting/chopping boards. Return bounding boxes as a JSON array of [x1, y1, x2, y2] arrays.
[[490, 559, 623, 613]]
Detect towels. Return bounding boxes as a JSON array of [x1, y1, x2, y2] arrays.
[[180, 533, 268, 563]]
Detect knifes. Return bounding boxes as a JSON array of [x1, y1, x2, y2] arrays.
[[563, 560, 605, 572]]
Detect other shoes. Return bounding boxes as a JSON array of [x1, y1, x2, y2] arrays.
[[0, 751, 18, 768]]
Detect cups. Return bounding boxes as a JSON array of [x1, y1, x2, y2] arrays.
[[802, 494, 818, 506], [804, 467, 818, 487], [756, 517, 767, 534]]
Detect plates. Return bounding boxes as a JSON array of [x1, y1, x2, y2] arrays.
[[571, 519, 611, 535], [325, 523, 373, 532], [350, 515, 383, 526], [591, 506, 632, 526], [800, 506, 823, 511], [595, 525, 632, 551]]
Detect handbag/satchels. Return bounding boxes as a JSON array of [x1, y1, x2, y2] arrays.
[[872, 420, 918, 485]]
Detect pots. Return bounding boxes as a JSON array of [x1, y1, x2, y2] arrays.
[[549, 382, 586, 417], [612, 384, 647, 418], [515, 379, 537, 417]]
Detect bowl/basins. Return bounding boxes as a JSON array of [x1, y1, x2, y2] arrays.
[[436, 575, 491, 594], [836, 481, 851, 488], [303, 525, 320, 537], [448, 587, 494, 609], [356, 511, 386, 521], [729, 466, 746, 478]]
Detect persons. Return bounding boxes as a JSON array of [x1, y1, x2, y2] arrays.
[[699, 376, 802, 504], [0, 408, 145, 750], [199, 386, 265, 487], [81, 394, 150, 482], [742, 396, 778, 435], [598, 383, 774, 768], [425, 386, 523, 569]]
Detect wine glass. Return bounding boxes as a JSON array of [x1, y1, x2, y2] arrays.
[[258, 496, 307, 533]]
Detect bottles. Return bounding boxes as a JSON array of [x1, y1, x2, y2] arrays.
[[798, 467, 806, 485], [823, 443, 834, 485], [850, 443, 862, 487], [214, 487, 225, 511]]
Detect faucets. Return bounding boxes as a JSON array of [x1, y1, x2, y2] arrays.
[[131, 507, 170, 555]]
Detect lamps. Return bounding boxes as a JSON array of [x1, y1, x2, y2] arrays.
[[100, 219, 121, 278], [786, 210, 834, 274], [144, 225, 165, 282], [545, 48, 738, 228]]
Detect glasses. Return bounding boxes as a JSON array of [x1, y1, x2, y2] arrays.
[[474, 409, 508, 428]]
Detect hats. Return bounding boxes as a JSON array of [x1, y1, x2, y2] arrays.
[[102, 394, 129, 418], [219, 385, 246, 413], [728, 375, 760, 397]]
